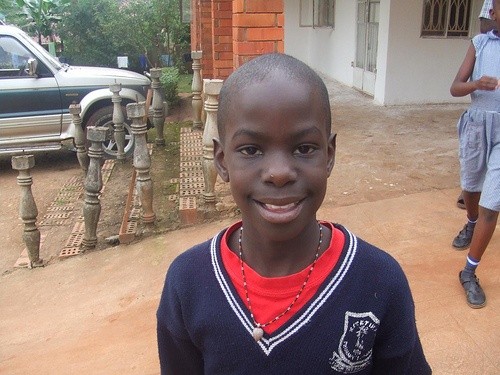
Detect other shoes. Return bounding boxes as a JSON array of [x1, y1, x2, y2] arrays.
[[457, 194, 466, 208]]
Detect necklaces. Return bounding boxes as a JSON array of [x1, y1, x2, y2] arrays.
[[238, 219, 323, 342]]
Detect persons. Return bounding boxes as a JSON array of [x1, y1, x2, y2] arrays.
[[155, 53, 435, 375], [450, 0, 500, 310]]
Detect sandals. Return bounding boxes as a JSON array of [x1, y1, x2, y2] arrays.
[[452, 222, 477, 250], [459, 270, 486, 309]]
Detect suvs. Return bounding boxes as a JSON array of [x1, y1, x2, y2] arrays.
[[0, 24, 152, 165]]
